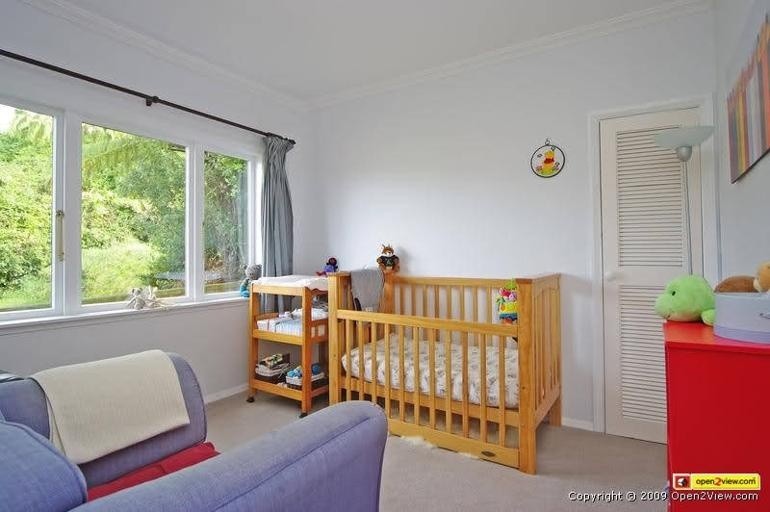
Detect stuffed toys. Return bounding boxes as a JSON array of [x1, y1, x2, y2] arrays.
[[316, 257, 339, 277], [240, 264, 263, 297], [144, 286, 167, 308], [495, 279, 518, 324], [376, 243, 400, 273], [126, 288, 146, 311], [752, 261, 770, 293], [654, 273, 760, 328]]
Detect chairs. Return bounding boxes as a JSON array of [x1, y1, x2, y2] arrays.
[[1, 352, 387, 511]]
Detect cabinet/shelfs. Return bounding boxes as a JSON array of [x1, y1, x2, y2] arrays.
[[662, 321, 770, 511], [246, 272, 327, 419]]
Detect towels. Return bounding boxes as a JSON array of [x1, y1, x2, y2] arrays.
[[25, 350, 191, 467]]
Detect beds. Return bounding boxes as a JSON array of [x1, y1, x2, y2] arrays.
[[328, 268, 564, 475]]
